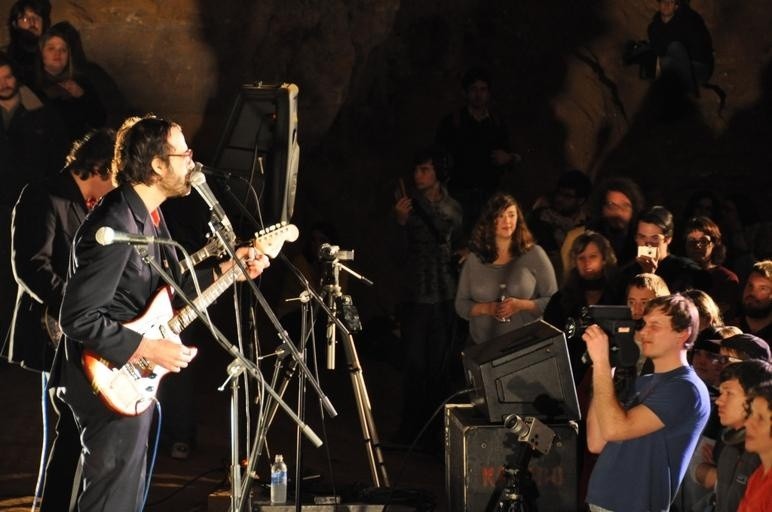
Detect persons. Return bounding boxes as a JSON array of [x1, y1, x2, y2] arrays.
[[0, 127, 117, 512], [0, 0, 127, 198], [397, 150, 772, 512], [624, 2, 715, 124], [262, 223, 348, 379], [434, 66, 518, 222], [46, 114, 270, 512]]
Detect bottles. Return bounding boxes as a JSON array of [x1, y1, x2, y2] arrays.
[[496, 284, 511, 323], [270, 454, 288, 506]]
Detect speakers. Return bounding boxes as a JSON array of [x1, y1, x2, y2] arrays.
[[461, 318, 582, 423], [444, 403, 579, 512]]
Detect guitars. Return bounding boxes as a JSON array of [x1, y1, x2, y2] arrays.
[[80, 219, 300, 416], [45, 223, 237, 348]]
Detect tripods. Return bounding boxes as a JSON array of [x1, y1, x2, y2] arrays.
[[492, 443, 533, 512], [227, 261, 390, 512]]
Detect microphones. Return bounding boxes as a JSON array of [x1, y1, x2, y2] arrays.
[[95, 226, 176, 246], [190, 171, 233, 232], [257, 149, 264, 174], [195, 162, 249, 184]]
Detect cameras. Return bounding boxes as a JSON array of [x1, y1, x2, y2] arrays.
[[638, 244, 657, 261], [504, 414, 556, 458], [319, 243, 354, 261]]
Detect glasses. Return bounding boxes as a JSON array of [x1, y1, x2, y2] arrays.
[[689, 238, 714, 246], [166, 148, 194, 160], [711, 354, 742, 366]]
